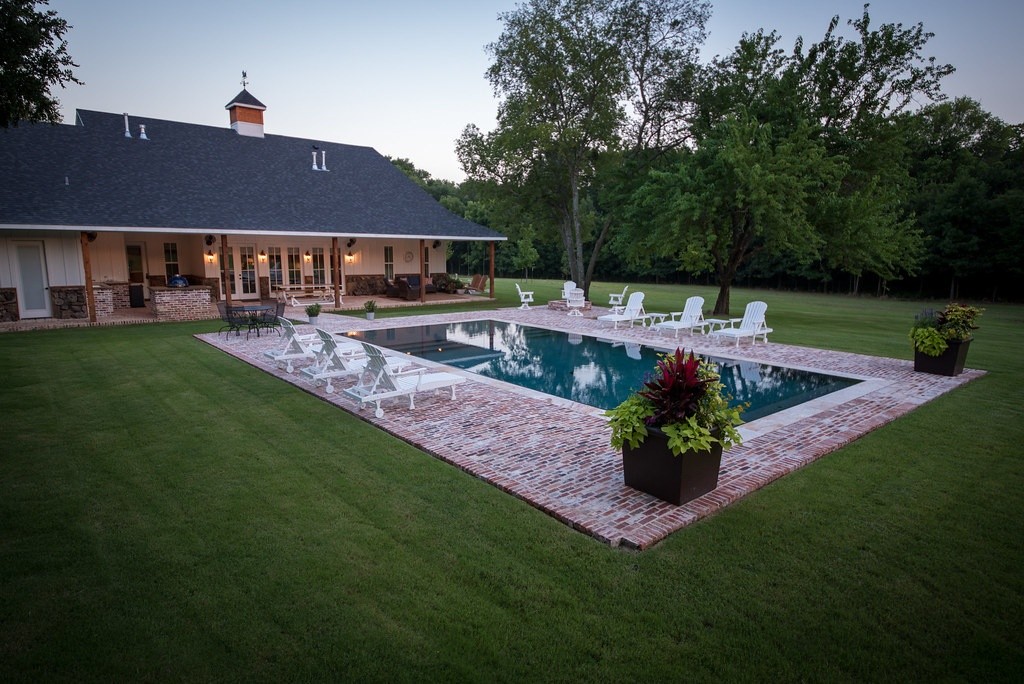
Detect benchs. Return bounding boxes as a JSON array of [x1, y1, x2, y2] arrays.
[[288, 291, 345, 307], [273, 289, 330, 305]]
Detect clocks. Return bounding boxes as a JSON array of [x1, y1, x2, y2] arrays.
[[403, 252, 413, 262]]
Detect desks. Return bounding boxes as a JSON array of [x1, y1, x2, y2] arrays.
[[361, 355, 412, 377], [705, 318, 731, 338], [228, 306, 274, 337], [644, 313, 670, 330], [311, 343, 361, 358], [271, 284, 343, 307]]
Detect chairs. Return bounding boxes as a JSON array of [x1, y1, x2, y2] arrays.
[[467, 274, 489, 294], [224, 303, 260, 343], [515, 283, 536, 311], [609, 285, 629, 313], [263, 316, 363, 374], [568, 333, 582, 345], [625, 342, 642, 360], [599, 291, 653, 329], [215, 302, 251, 336], [300, 327, 412, 395], [566, 288, 585, 317], [261, 302, 287, 337], [728, 361, 762, 384], [343, 340, 468, 420], [715, 301, 774, 348], [597, 338, 624, 348], [657, 295, 709, 337], [561, 280, 577, 300]]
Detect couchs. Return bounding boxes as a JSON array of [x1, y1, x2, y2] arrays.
[[383, 276, 398, 298], [398, 280, 420, 302], [397, 276, 437, 294]]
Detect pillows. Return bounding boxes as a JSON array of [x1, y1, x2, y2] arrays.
[[424, 278, 428, 286], [428, 277, 433, 285], [400, 278, 407, 281]]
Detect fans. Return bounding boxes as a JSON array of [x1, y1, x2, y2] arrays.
[[204, 234, 217, 246], [347, 238, 356, 247], [433, 240, 442, 249], [79, 231, 97, 244]]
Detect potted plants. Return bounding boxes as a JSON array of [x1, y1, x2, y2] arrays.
[[600, 346, 750, 506], [304, 301, 322, 326], [908, 302, 987, 376], [456, 278, 466, 295], [363, 300, 378, 320]]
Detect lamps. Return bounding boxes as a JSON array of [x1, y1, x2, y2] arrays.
[[207, 249, 214, 263], [348, 251, 353, 263], [305, 250, 312, 264], [259, 250, 267, 263]]
